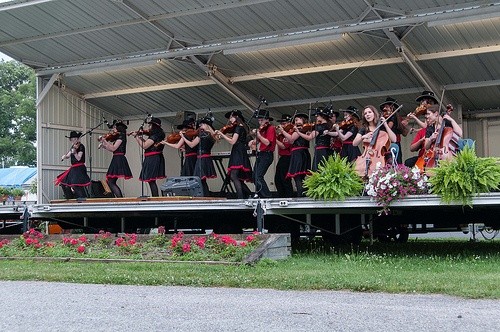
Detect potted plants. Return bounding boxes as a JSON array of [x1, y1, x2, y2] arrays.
[[0, 188, 26, 203]]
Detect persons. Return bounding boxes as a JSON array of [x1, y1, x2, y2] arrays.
[[292, 107, 340, 173], [160, 118, 198, 176], [215, 109, 255, 198], [378, 97, 408, 164], [55, 131, 91, 198], [248, 109, 276, 198], [408, 89, 438, 134], [101, 120, 134, 197], [353, 105, 397, 153], [133, 118, 166, 197], [323, 106, 361, 162], [405, 105, 461, 168], [179, 117, 217, 195], [275, 113, 312, 195]]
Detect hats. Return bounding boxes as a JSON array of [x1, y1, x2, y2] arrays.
[[415, 91, 438, 104], [343, 107, 360, 119], [149, 118, 161, 127], [380, 97, 400, 112], [225, 110, 244, 120], [64, 131, 82, 138], [312, 107, 332, 118], [277, 114, 293, 122], [200, 117, 212, 126], [255, 110, 274, 121], [333, 111, 340, 118]]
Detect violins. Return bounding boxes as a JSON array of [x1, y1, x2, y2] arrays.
[[251, 122, 271, 136], [70, 141, 81, 153], [273, 121, 322, 138], [97, 131, 122, 142], [401, 104, 430, 125], [332, 118, 354, 131], [127, 129, 156, 137], [151, 127, 205, 151], [211, 121, 237, 138], [378, 111, 394, 118]]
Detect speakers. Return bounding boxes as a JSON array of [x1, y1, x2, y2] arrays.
[[160, 176, 204, 197]]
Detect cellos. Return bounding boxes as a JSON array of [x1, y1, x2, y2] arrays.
[[411, 103, 461, 194], [352, 109, 392, 197]]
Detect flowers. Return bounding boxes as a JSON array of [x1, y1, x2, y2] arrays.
[[366, 162, 429, 216]]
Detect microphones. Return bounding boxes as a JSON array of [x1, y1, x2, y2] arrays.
[[103, 116, 111, 129], [261, 95, 268, 105], [330, 102, 333, 117], [147, 112, 153, 119], [208, 108, 215, 121]]
